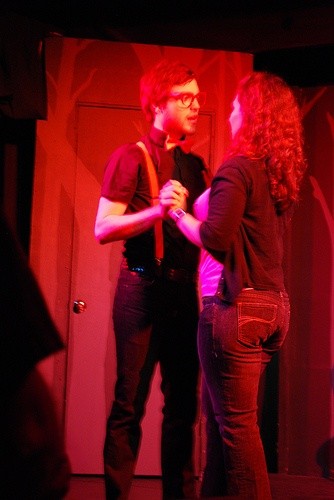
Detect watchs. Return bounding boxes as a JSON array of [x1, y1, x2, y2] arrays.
[[171, 207, 189, 224]]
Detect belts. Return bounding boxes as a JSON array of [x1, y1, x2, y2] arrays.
[[128, 264, 187, 281]]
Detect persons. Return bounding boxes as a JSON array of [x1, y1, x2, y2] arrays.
[[0, 208, 73, 499], [159, 71, 308, 500], [91, 58, 213, 499]]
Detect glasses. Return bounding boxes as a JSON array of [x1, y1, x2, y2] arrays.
[[167, 90, 206, 108]]
